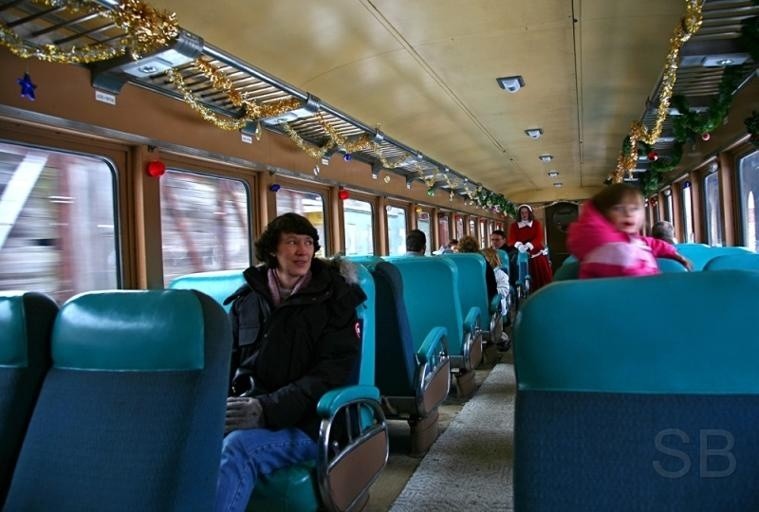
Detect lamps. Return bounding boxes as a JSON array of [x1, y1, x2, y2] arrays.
[[497, 72, 529, 94], [524, 126, 546, 142], [539, 152, 567, 192]]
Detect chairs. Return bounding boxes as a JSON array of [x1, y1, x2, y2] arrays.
[[2, 245, 757, 512]]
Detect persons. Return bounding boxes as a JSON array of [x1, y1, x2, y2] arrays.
[[406, 229, 519, 353], [563, 182, 691, 280], [506, 203, 553, 296], [651, 220, 679, 245], [214, 215, 365, 512]]
